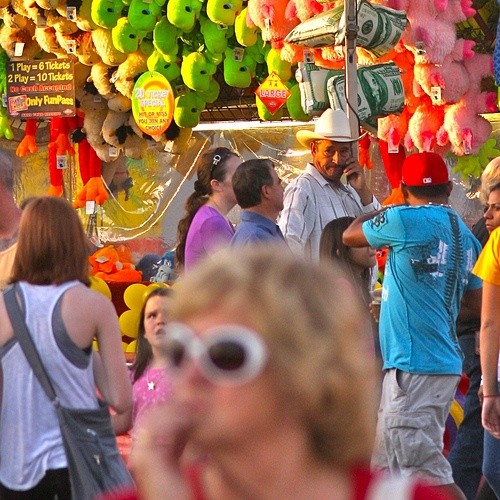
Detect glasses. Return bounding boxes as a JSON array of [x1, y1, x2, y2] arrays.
[[158, 321, 279, 389], [210, 147, 230, 179]]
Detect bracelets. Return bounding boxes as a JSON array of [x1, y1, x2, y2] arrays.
[[482, 393, 500, 398]]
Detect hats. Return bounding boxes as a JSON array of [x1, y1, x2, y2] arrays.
[[401, 151, 448, 186], [296, 108, 367, 150]]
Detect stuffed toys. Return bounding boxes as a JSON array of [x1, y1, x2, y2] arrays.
[[85, 244, 179, 284], [0, 0, 500, 210]]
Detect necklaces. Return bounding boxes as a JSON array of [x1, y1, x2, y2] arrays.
[[146, 364, 165, 391]]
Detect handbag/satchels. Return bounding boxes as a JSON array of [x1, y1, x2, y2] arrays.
[[2, 284, 137, 500]]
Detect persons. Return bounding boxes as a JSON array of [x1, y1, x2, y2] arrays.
[[127, 243, 384, 500], [0, 195, 133, 500], [228, 158, 291, 250], [126, 286, 185, 440], [0, 196, 39, 290], [277, 107, 384, 295], [318, 216, 384, 373], [341, 151, 500, 500], [176, 146, 243, 272]]
